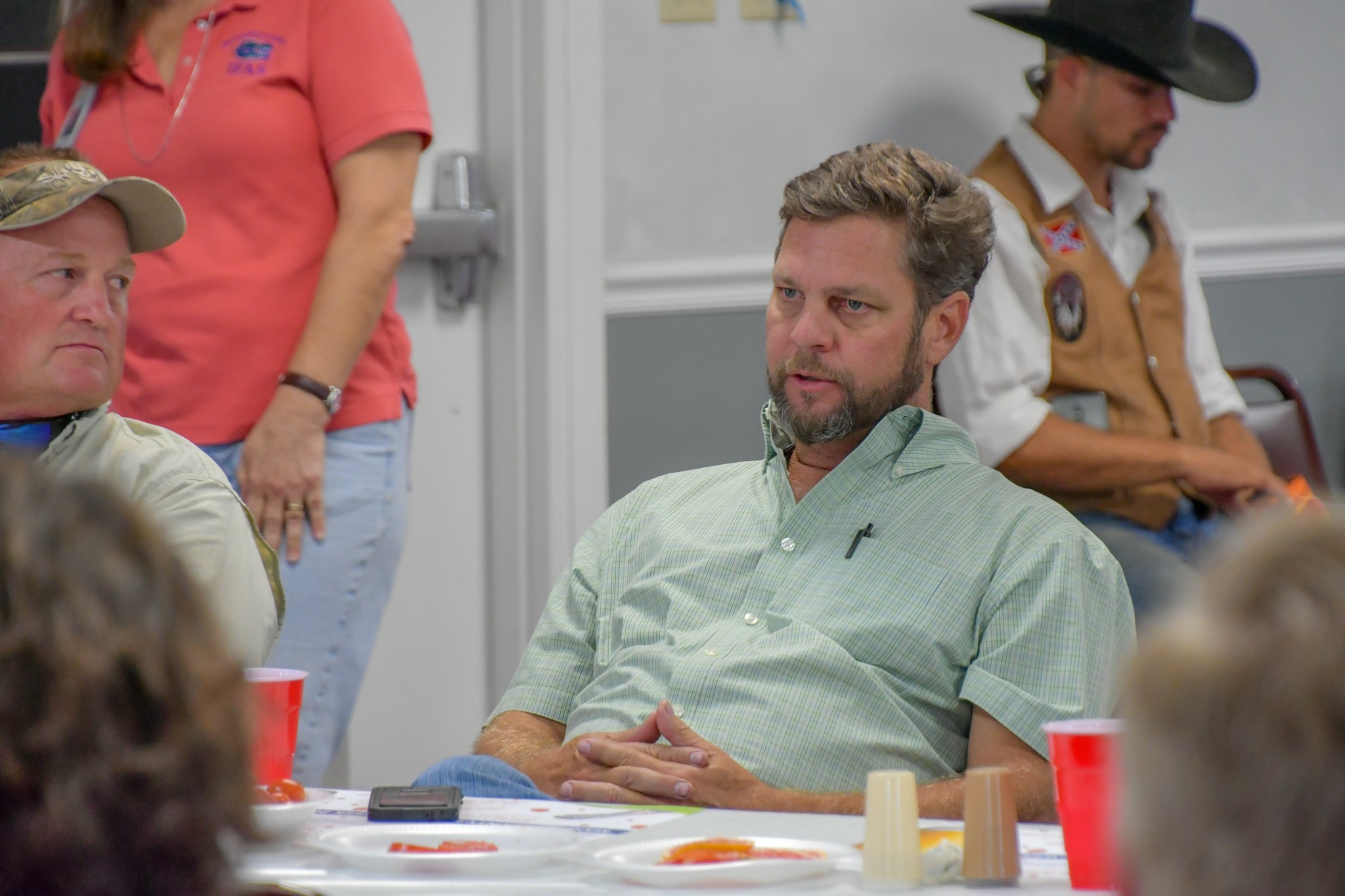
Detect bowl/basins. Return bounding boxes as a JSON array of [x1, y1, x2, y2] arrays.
[[234, 788, 340, 854]]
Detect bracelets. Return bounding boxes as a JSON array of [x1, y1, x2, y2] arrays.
[[278, 369, 342, 414]]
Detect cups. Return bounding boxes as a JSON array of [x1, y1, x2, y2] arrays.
[[862, 769, 921, 893], [1044, 708, 1136, 890], [962, 764, 1021, 887], [245, 663, 311, 791]]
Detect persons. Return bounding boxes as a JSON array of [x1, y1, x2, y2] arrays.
[[0, 133, 281, 674], [404, 136, 1138, 829], [0, 446, 303, 896], [38, 0, 439, 790], [934, 0, 1294, 643]]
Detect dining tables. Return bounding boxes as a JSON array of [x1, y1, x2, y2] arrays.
[[221, 780, 1112, 895]]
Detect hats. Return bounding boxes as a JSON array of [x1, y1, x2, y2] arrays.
[[1, 162, 185, 255], [970, 1, 1261, 104]]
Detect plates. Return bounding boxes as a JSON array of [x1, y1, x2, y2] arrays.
[[315, 823, 578, 882], [589, 836, 854, 888]]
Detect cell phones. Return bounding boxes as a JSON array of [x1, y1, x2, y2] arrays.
[[365, 785, 462, 822]]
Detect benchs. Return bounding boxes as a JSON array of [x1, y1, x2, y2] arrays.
[[1227, 359, 1338, 506]]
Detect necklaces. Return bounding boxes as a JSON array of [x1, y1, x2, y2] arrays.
[[109, 6, 218, 165]]
